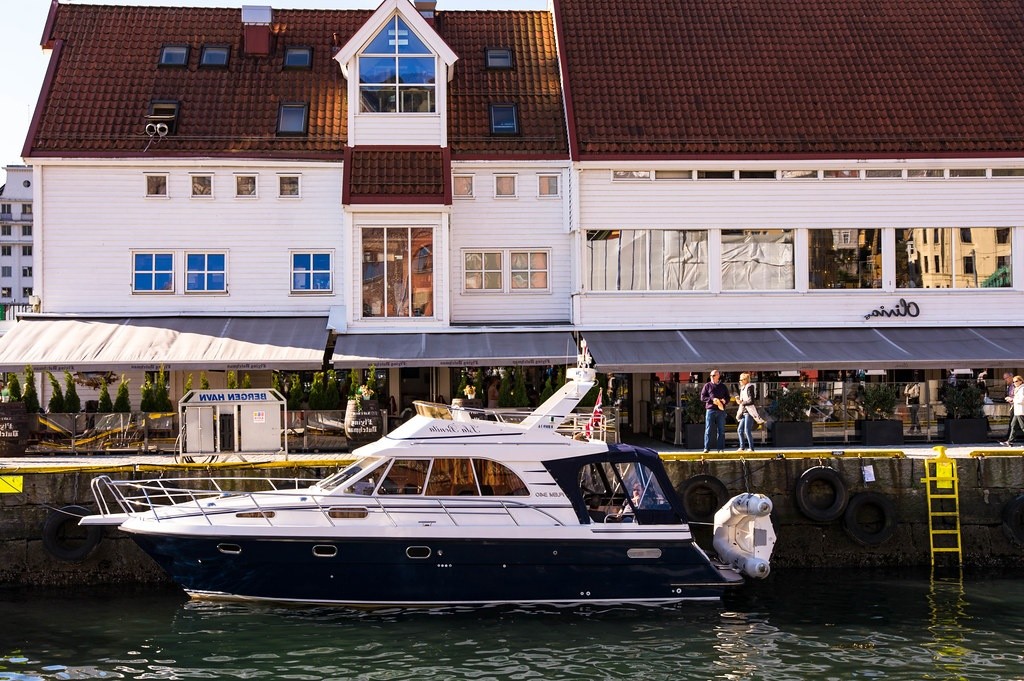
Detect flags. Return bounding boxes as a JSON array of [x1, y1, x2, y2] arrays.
[[586, 393, 603, 436]]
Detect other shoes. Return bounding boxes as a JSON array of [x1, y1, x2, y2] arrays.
[[906, 430, 914, 434], [914, 430, 922, 434], [736, 448, 744, 451], [1000, 441, 1013, 448], [704, 448, 709, 453], [718, 448, 724, 453], [747, 448, 754, 452]]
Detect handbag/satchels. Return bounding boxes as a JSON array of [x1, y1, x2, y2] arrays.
[[908, 396, 919, 404]]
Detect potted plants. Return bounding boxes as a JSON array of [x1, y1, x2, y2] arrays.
[[764, 384, 813, 446], [666, 387, 717, 449], [937, 381, 988, 443], [854, 383, 904, 447]]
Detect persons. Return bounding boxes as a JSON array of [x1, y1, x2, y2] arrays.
[[700, 370, 730, 453], [903, 382, 922, 434], [996, 371, 1024, 446], [616, 483, 655, 523], [486, 378, 500, 408], [734, 373, 756, 452]]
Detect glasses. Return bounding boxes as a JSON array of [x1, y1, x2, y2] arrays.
[[633, 488, 644, 492], [710, 375, 718, 378]]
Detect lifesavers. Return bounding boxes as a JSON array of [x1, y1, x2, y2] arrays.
[[676, 474, 729, 527], [841, 491, 899, 547], [1000, 494, 1024, 550], [794, 464, 849, 524], [43, 505, 104, 564]]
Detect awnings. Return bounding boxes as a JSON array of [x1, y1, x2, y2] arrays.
[[580, 327, 1024, 374], [0, 317, 330, 373], [331, 332, 578, 369]]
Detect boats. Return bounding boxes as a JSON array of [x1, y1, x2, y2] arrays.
[[77, 338, 779, 614]]
